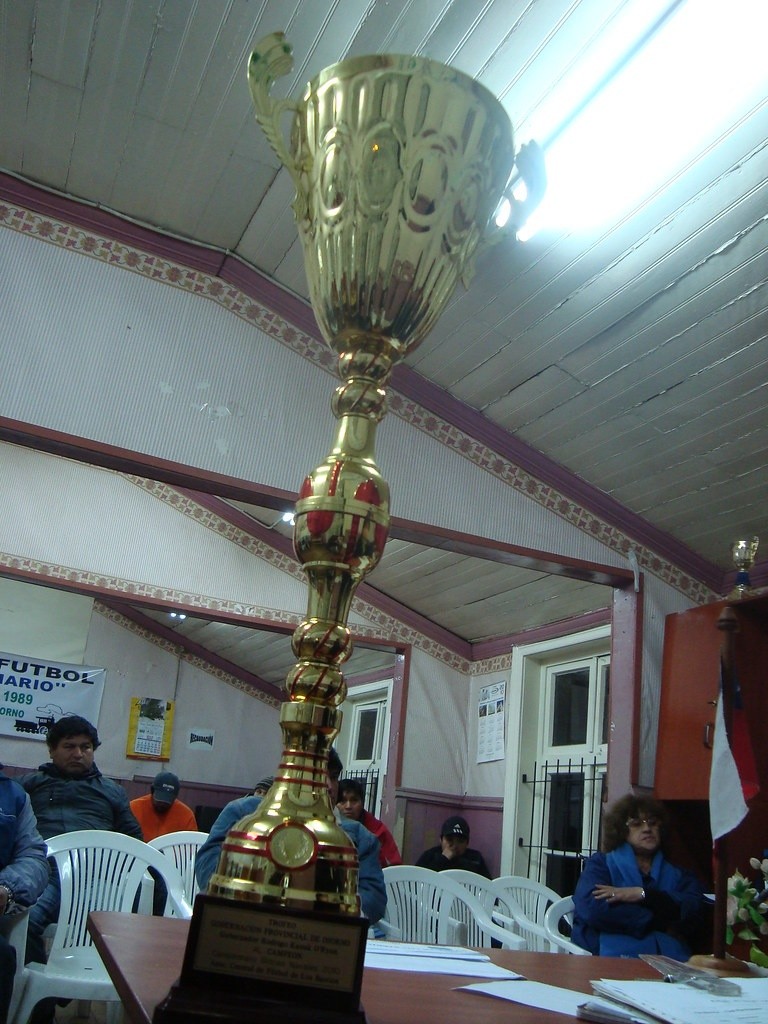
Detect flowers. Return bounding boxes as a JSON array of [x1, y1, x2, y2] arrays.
[[726, 858, 768, 967]]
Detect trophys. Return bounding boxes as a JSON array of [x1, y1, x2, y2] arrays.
[[151, 32, 548, 1024], [716, 536, 763, 602]]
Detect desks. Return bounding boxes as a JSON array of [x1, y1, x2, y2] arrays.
[[89, 912, 664, 1023]]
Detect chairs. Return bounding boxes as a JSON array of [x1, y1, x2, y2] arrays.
[[8, 830, 593, 1024]]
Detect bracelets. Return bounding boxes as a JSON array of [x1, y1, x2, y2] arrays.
[[642, 891, 645, 898]]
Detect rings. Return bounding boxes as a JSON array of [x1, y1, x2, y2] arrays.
[[612, 892, 614, 897]]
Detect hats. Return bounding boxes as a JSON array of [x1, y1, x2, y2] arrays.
[[254, 776, 274, 791], [442, 816, 469, 840], [152, 772, 180, 805]]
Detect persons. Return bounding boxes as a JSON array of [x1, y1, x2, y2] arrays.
[[315, 859, 344, 912], [355, 528, 372, 558], [572, 793, 703, 961], [129, 772, 198, 843], [297, 536, 310, 551], [254, 777, 274, 797], [328, 535, 344, 548], [0, 762, 52, 1024], [194, 745, 387, 926], [409, 815, 502, 949], [336, 779, 403, 867], [12, 716, 167, 1024]]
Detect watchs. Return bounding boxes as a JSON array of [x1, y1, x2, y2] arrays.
[[0, 885, 15, 914]]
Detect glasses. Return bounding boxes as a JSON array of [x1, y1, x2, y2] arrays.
[[625, 816, 663, 830]]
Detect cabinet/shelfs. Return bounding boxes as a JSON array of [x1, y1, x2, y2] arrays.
[[655, 593, 768, 800]]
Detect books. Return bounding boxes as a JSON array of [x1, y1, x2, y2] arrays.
[[577, 1003, 636, 1024]]
